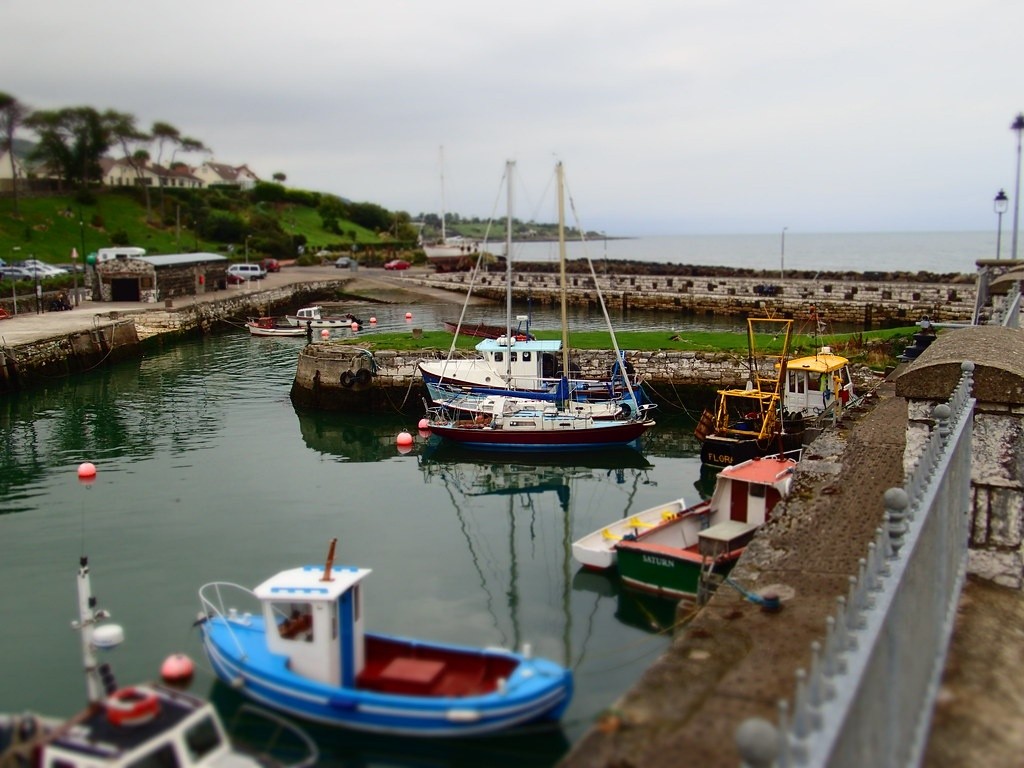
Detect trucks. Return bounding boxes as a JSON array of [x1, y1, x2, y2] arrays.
[[98, 247, 146, 265]]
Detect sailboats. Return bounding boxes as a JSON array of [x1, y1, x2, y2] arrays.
[[412, 150, 658, 455]]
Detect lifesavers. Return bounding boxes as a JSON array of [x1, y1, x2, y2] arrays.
[[109, 686, 162, 724], [340, 370, 355, 387], [356, 368, 371, 385]]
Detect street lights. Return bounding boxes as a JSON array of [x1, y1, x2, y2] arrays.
[[246, 235, 252, 265], [1010, 114, 1024, 259], [992, 189, 1008, 260]]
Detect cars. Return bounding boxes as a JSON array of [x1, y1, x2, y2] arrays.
[[0, 259, 86, 281], [384, 260, 410, 271], [225, 270, 244, 284], [318, 251, 332, 256], [335, 257, 350, 268], [263, 259, 279, 272], [259, 264, 267, 276]]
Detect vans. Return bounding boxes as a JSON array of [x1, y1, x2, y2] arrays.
[[228, 265, 261, 281]]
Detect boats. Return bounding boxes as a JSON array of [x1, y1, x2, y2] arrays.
[[571, 498, 688, 573], [285, 306, 353, 328], [247, 317, 306, 337], [609, 445, 803, 605], [421, 145, 481, 270], [194, 537, 575, 743], [444, 322, 535, 341], [1, 556, 319, 768], [693, 347, 857, 473]]
